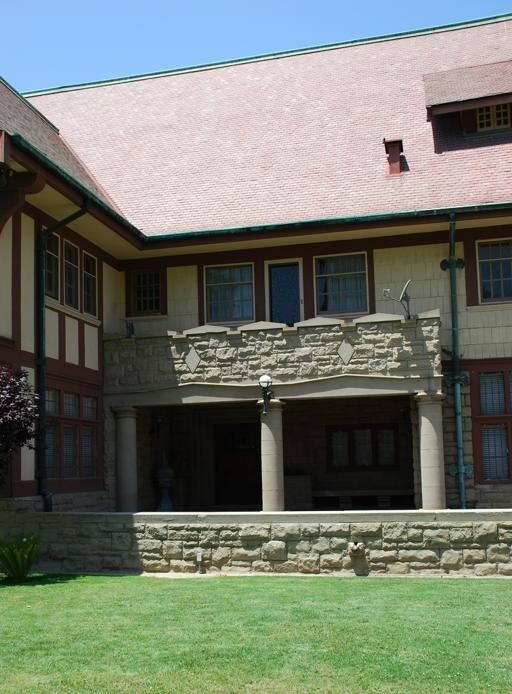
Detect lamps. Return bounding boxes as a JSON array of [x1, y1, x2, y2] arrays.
[[257, 377, 274, 417]]
